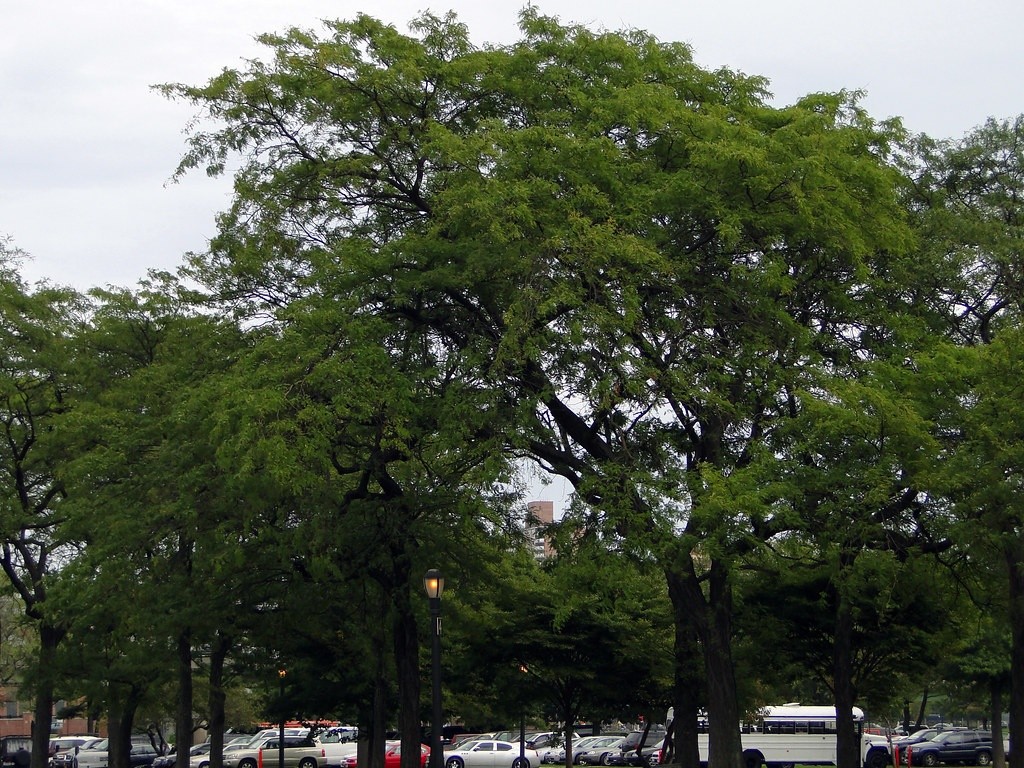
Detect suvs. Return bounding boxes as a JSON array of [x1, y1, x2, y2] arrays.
[[906, 729, 994, 766], [221, 735, 327, 768]]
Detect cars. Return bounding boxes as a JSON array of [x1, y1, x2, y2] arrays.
[[0, 720, 1010, 768]]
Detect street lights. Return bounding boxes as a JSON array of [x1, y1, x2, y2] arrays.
[[424, 563, 446, 768], [275, 666, 288, 766]]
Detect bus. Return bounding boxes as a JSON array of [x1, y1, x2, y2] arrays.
[[664, 703, 897, 768]]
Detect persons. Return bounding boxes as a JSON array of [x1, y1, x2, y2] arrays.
[[13, 747, 31, 768]]
